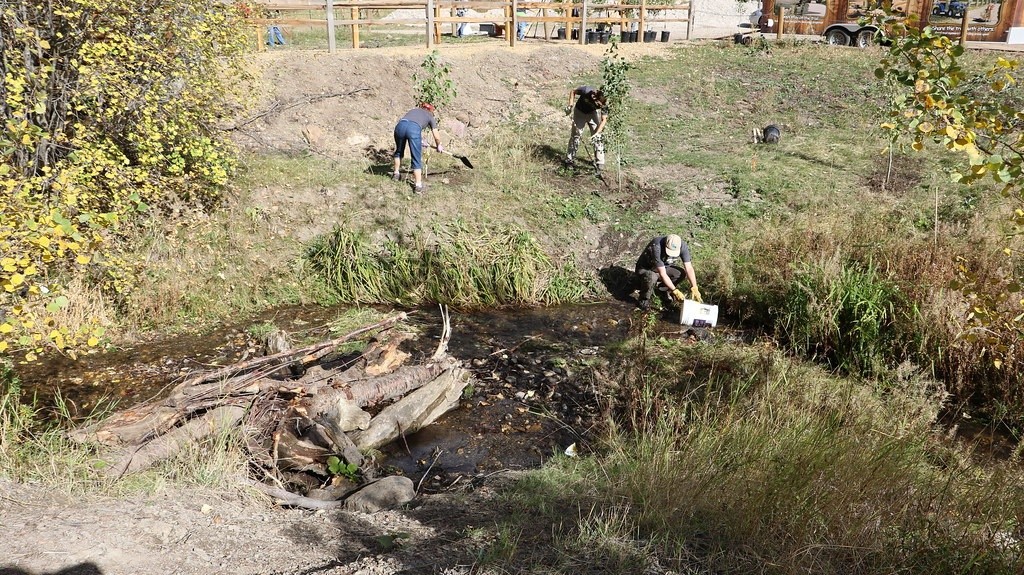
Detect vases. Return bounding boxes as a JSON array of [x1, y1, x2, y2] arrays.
[[763, 124, 780, 144]]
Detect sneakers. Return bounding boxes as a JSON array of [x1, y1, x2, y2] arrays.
[[412, 181, 424, 195], [391, 171, 400, 182]]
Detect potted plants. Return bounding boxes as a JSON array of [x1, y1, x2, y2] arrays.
[[548, 0, 683, 43], [732, 0, 748, 44]]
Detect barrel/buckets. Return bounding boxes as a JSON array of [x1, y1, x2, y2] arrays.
[[572, 8, 579, 17], [744, 36, 753, 46], [734, 33, 743, 44], [557, 28, 670, 44], [679, 298, 719, 328], [763, 126, 779, 144]]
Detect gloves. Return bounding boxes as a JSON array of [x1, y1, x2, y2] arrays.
[[690, 286, 704, 304], [672, 289, 685, 302]]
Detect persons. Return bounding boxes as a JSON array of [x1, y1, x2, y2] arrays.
[[393, 101, 442, 192], [985, 1, 994, 22], [635, 235, 703, 310], [456, 0, 467, 38], [266, 10, 284, 48], [563, 87, 610, 174]]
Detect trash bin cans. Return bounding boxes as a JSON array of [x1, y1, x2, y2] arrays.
[[599, 31, 609, 44], [621, 31, 629, 43], [589, 31, 599, 44], [732, 33, 753, 47], [630, 30, 657, 43], [763, 125, 780, 144], [661, 31, 670, 42], [558, 28, 603, 45]]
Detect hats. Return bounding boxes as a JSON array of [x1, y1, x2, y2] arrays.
[[422, 102, 436, 112], [591, 89, 609, 109], [665, 233, 682, 258]]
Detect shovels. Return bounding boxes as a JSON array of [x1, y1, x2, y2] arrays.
[[565, 106, 611, 188], [426, 143, 475, 170]]
